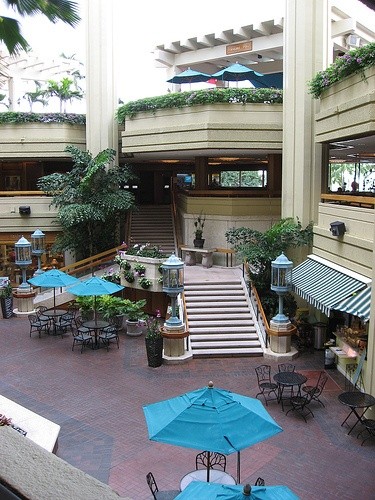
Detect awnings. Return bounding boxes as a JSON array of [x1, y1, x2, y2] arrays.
[[333, 285, 370, 319], [283, 254, 366, 318]]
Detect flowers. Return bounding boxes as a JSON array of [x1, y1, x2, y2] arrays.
[[0, 280, 12, 297], [144, 310, 164, 337], [101, 242, 167, 290]]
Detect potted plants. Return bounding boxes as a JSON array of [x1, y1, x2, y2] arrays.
[[193, 210, 206, 248], [69, 295, 147, 336]]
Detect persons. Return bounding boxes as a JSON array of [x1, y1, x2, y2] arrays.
[[351, 177, 359, 192], [341, 182, 347, 191]]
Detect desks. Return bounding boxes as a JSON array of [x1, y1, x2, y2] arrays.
[[273, 372, 307, 412], [179, 244, 234, 267], [337, 392, 375, 435], [179, 469, 236, 493], [43, 310, 68, 335], [83, 320, 110, 351]]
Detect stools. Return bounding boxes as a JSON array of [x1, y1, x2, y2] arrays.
[[357, 419, 375, 446]]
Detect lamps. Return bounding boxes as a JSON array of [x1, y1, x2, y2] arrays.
[[329, 221, 346, 236], [19, 206, 30, 215]]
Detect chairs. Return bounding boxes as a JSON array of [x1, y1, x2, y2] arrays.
[[28, 305, 119, 355], [146, 451, 265, 500], [255, 363, 328, 424]]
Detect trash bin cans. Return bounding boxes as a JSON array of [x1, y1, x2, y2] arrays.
[[313, 322, 328, 350]]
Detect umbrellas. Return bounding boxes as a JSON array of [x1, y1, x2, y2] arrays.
[[211, 61, 264, 88], [66, 276, 126, 345], [166, 67, 212, 90], [141, 381, 284, 482], [174, 480, 301, 500], [27, 268, 80, 334], [249, 71, 283, 89]]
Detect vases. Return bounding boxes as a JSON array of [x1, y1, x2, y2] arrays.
[[124, 266, 130, 270], [141, 285, 150, 289], [145, 337, 163, 368], [0, 297, 14, 319], [138, 269, 145, 277], [158, 268, 163, 274], [126, 278, 134, 283], [115, 278, 120, 281]]
